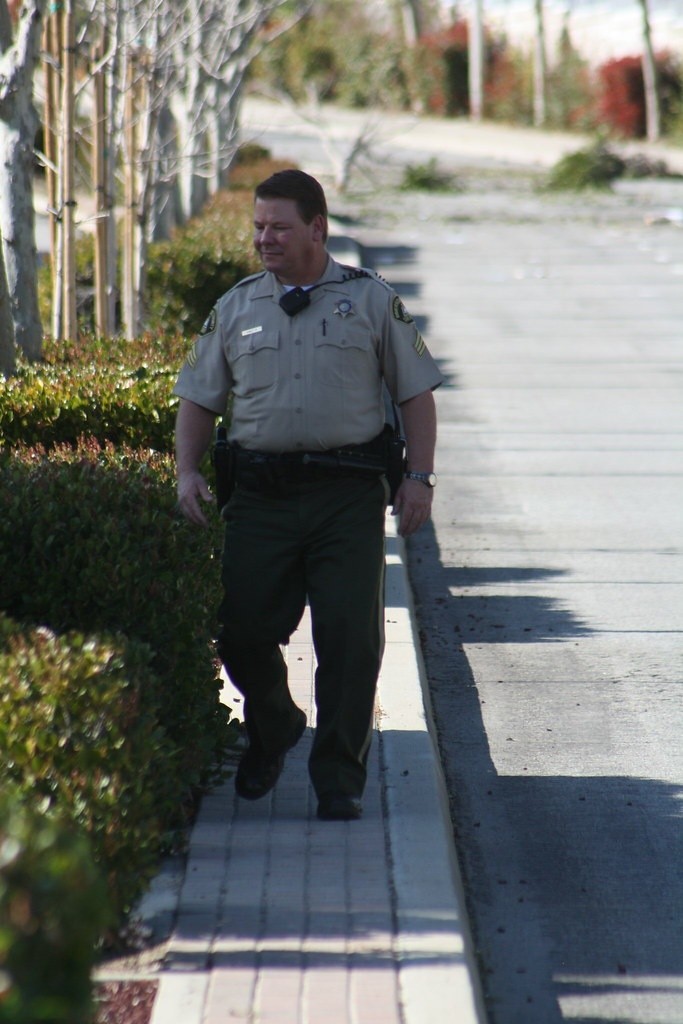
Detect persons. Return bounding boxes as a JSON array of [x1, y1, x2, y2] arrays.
[[172, 168, 445, 821]]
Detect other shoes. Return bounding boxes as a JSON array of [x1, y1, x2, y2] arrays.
[[234, 702, 306, 800], [317, 794, 362, 819]]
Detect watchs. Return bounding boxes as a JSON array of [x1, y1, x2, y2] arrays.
[[401, 472, 437, 488]]
